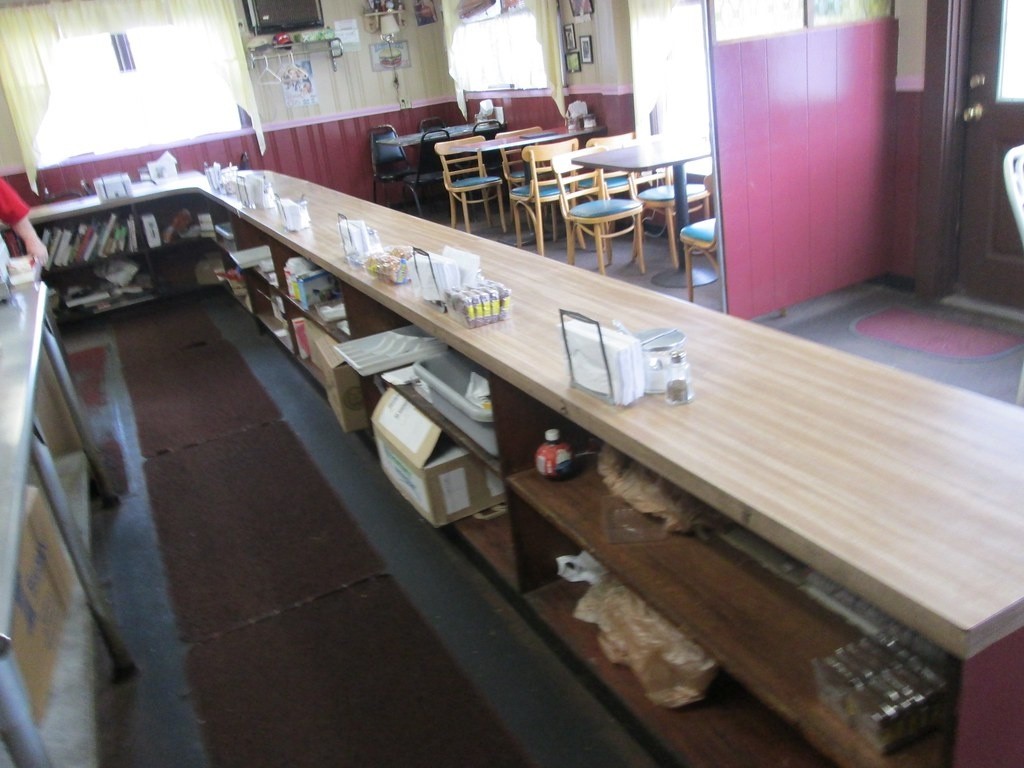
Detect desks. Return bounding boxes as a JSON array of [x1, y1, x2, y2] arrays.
[[375, 120, 507, 221], [571, 139, 718, 288], [451, 123, 606, 247]]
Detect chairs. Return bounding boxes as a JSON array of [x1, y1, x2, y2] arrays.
[[369, 116, 790, 320]]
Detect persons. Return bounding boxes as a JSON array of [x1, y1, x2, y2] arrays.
[[0, 177, 49, 267]]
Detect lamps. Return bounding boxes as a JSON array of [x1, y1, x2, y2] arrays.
[[382, 14, 404, 92]]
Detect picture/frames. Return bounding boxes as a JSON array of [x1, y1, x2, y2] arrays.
[[579, 35, 592, 64], [565, 53, 581, 74], [569, 0, 594, 17], [564, 23, 577, 51]]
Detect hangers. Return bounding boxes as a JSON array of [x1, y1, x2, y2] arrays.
[[257, 55, 282, 86], [282, 51, 308, 82]]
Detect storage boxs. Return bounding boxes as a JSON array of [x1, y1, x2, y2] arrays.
[[368, 385, 508, 530], [314, 334, 372, 433], [9, 484, 85, 720]]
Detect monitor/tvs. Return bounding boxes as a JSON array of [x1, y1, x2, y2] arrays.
[[246, 0, 324, 36]]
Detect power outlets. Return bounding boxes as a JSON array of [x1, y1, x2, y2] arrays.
[[399, 96, 410, 107]]
